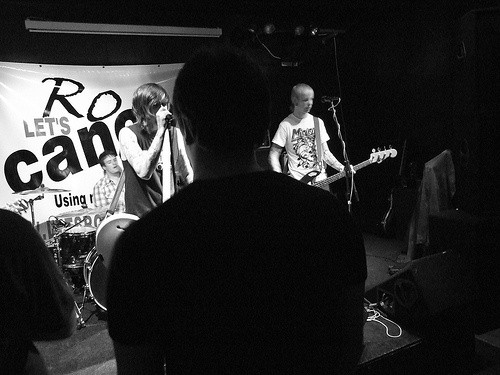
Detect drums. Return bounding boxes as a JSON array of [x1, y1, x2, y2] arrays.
[[59, 226, 98, 260], [62, 262, 92, 288], [82, 244, 109, 312]]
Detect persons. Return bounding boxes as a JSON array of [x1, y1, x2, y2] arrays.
[[106, 46, 368, 375], [92, 82, 357, 219], [0, 208, 76, 375]]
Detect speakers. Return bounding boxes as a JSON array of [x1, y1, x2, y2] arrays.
[[366, 251, 479, 336]]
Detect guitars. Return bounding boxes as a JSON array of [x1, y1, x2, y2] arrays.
[[299, 142, 399, 188], [377, 137, 414, 237]]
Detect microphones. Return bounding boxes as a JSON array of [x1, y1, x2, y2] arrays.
[[321, 95, 341, 103], [166, 114, 173, 123]]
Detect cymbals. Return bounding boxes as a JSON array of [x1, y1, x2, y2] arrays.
[[11, 183, 71, 196]]
